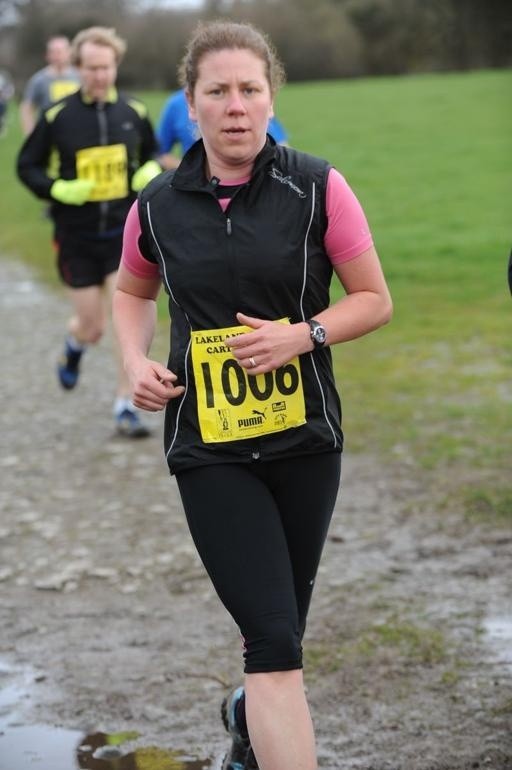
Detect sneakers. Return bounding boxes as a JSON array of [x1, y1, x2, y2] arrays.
[[57, 337, 84, 391], [112, 407, 151, 437], [219, 685, 259, 770]]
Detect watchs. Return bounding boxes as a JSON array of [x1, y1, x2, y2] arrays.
[[305, 319, 328, 351]]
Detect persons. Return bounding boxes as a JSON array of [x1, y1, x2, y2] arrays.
[[12, 28, 163, 438], [19, 36, 86, 251], [0, 70, 14, 136], [118, 21, 394, 769], [152, 69, 291, 171]]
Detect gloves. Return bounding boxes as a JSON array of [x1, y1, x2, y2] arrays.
[[50, 178, 94, 207], [131, 159, 164, 192]]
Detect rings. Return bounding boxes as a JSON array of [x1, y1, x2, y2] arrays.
[[248, 358, 256, 368]]
